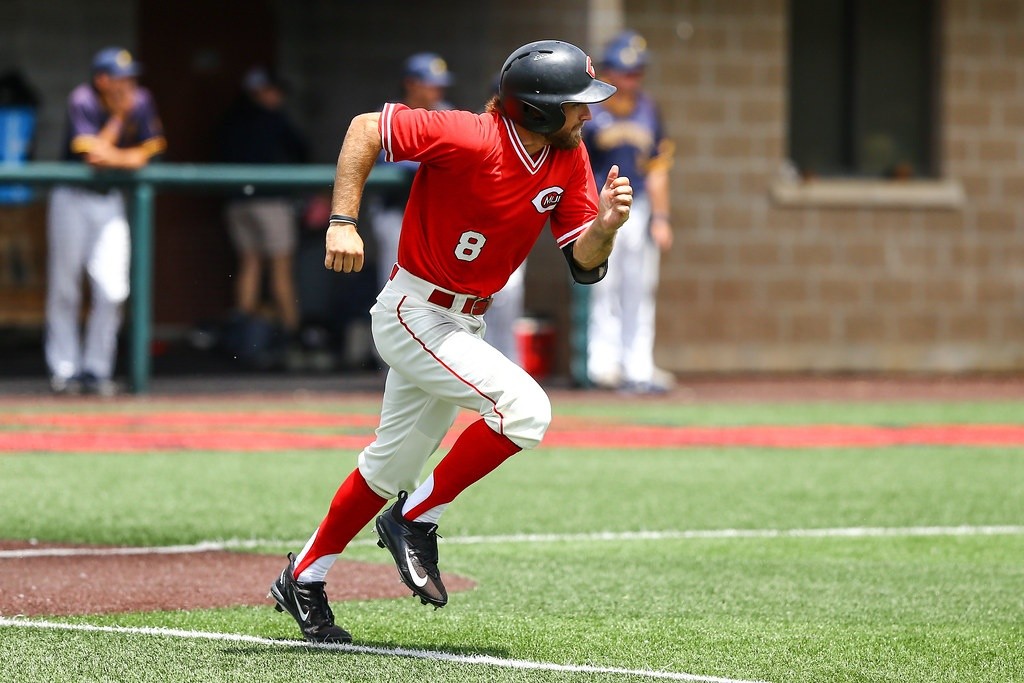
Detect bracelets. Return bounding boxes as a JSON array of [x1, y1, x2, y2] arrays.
[[329, 215, 358, 228], [650, 212, 672, 224]]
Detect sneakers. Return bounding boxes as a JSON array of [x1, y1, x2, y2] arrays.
[[375, 490, 448, 612], [268, 550, 351, 644]]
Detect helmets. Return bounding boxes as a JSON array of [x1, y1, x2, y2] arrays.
[[498, 39, 618, 133]]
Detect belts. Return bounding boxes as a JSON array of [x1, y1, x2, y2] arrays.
[[389, 263, 494, 315]]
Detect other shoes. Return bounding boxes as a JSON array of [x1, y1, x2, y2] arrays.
[[52, 372, 122, 397]]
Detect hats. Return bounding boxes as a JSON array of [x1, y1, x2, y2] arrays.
[[599, 29, 648, 71], [404, 51, 456, 89], [90, 46, 140, 80]]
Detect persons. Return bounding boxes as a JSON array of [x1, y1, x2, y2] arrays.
[[0, 67, 41, 286], [370, 55, 453, 291], [44, 48, 167, 399], [580, 31, 678, 394], [268, 39, 633, 645], [218, 61, 330, 333]]
